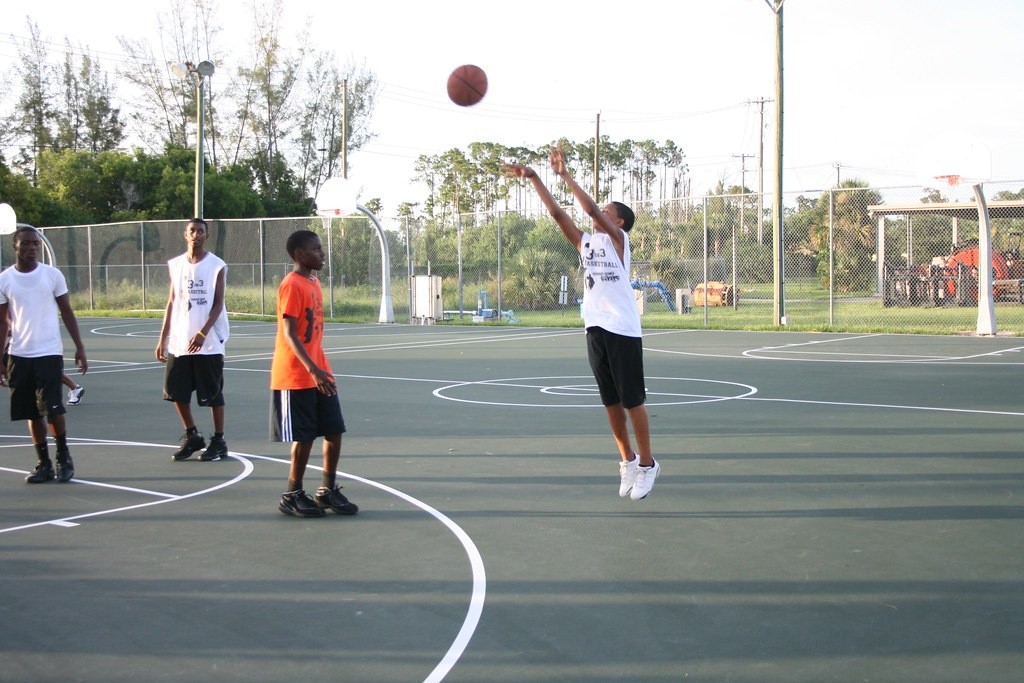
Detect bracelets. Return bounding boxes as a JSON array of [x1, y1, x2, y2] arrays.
[[197, 331, 206, 339]]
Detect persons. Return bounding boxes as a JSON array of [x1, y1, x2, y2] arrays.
[[0, 227, 87, 484], [499, 143, 660, 501], [156, 218, 230, 462], [269, 231, 359, 518], [0, 309, 85, 406]]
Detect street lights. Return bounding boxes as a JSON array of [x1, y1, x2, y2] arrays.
[[171, 59, 215, 219]]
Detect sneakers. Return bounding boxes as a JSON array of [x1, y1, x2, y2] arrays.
[[67, 384, 85, 406], [314, 484, 359, 514], [56, 451, 74, 481], [278, 488, 326, 517], [630, 455, 660, 501], [24, 459, 55, 484], [172, 432, 206, 461], [198, 434, 227, 461], [618, 451, 640, 497]]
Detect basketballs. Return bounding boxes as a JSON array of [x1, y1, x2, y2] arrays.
[[446, 64, 489, 107]]
